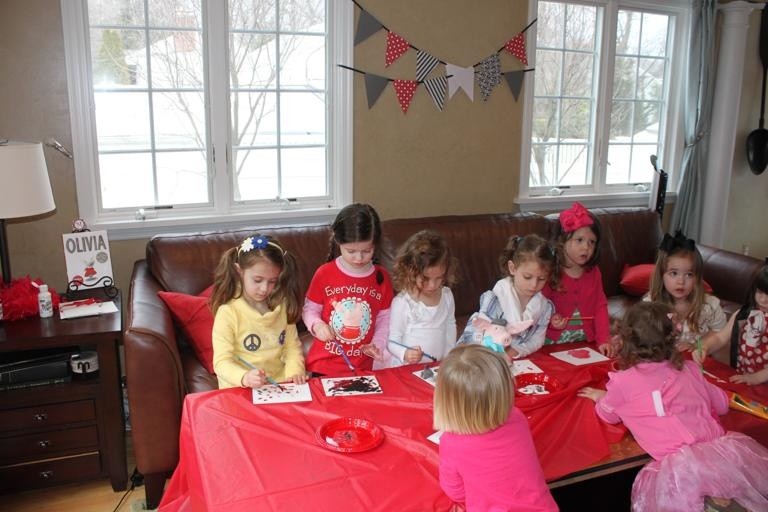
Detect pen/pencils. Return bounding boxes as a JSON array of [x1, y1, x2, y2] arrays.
[[697, 335, 704, 376], [547, 317, 595, 320]]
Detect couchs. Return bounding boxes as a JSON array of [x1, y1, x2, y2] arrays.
[[123, 209, 768, 510]]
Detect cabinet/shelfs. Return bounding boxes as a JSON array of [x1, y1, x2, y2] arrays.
[[0, 291, 129, 492]]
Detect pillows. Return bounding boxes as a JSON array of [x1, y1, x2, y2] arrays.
[[619, 260, 713, 296], [155, 281, 216, 379]]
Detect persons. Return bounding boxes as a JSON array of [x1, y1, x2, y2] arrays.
[[541, 199, 619, 361], [608, 229, 728, 355], [574, 300, 768, 512], [424, 343, 561, 512], [458, 232, 571, 363], [690, 256, 768, 387], [205, 232, 309, 390], [385, 229, 458, 370], [299, 203, 395, 379]]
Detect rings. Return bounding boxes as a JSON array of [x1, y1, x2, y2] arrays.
[[611, 351, 614, 354], [614, 352, 618, 355]]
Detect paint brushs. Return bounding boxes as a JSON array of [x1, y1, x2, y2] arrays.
[[238, 357, 284, 392], [332, 339, 355, 372], [388, 340, 437, 362]]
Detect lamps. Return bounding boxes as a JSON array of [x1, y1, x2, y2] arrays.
[[0, 138, 57, 285]]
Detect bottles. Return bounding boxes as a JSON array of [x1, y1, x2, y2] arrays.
[[31, 280, 54, 320]]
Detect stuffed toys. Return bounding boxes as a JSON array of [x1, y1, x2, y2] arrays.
[[472, 318, 535, 348]]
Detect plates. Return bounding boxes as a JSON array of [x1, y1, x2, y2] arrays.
[[510, 372, 564, 402], [312, 416, 385, 454]]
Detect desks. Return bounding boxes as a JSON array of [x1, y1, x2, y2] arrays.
[[153, 343, 763, 510]]
